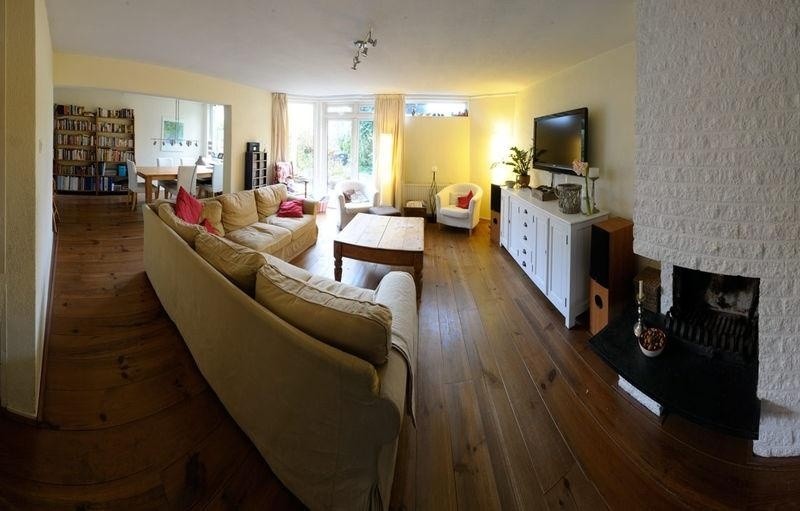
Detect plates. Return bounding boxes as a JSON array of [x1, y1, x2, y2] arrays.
[[499, 185, 611, 331], [403, 206, 428, 230], [244, 142, 267, 191], [52, 102, 137, 212]]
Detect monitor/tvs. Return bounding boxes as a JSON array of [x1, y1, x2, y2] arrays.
[[53, 103, 134, 192]]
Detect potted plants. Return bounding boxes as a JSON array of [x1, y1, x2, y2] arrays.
[[159, 117, 186, 152]]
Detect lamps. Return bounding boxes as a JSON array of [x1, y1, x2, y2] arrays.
[[125, 159, 157, 211], [200, 164, 223, 199], [434, 181, 483, 236], [155, 156, 178, 199], [52, 179, 62, 233], [274, 161, 310, 200], [164, 163, 198, 200], [339, 182, 382, 231]]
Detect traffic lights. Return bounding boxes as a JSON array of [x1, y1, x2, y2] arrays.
[[199, 219, 222, 238], [344, 185, 360, 200], [351, 193, 369, 203], [276, 199, 306, 219], [457, 190, 473, 210], [449, 192, 467, 206], [174, 185, 204, 225]]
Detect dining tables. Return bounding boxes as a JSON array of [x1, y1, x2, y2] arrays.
[[490, 142, 535, 188]]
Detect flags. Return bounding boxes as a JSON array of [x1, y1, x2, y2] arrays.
[[554, 181, 582, 215], [579, 182, 595, 217]]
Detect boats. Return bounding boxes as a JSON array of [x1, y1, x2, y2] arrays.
[[491, 184, 501, 247], [588, 216, 635, 338]]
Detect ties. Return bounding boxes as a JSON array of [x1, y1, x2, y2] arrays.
[[533, 107, 588, 177]]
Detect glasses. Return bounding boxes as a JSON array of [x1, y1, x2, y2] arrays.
[[349, 21, 377, 73], [148, 97, 201, 149], [188, 155, 207, 199]]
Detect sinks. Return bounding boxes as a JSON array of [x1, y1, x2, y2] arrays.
[[142, 183, 418, 510]]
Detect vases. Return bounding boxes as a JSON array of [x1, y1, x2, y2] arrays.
[[572, 158, 593, 217]]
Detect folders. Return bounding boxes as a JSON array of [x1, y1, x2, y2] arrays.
[[406, 183, 449, 214]]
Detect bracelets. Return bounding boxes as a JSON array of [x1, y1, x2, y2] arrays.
[[638, 327, 667, 357]]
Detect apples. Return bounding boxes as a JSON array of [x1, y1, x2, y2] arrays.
[[638, 280, 644, 299], [589, 168, 599, 178]]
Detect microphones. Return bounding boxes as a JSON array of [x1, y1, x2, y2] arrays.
[[368, 205, 400, 217]]
